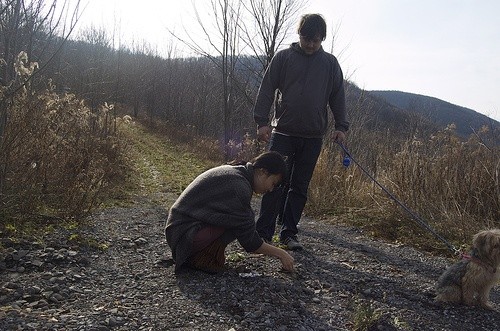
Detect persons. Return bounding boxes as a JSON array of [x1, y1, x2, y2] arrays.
[[164, 151, 298, 274], [252, 13, 350, 251]]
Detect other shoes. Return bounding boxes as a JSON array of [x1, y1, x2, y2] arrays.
[[190, 256, 225, 274], [285, 233, 304, 250]]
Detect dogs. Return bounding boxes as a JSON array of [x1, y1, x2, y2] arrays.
[[435, 229, 500, 315]]
